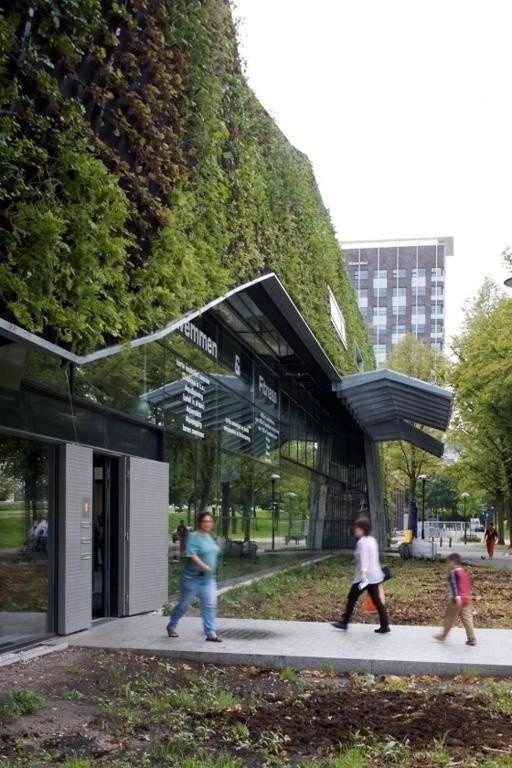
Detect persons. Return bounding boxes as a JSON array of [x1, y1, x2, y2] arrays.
[[434, 554, 479, 646], [483, 523, 499, 560], [331, 518, 393, 633], [177, 520, 188, 561], [25, 514, 48, 551], [167, 512, 223, 643]]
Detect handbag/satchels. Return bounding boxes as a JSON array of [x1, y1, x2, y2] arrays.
[[363, 584, 385, 613], [383, 564, 393, 579]]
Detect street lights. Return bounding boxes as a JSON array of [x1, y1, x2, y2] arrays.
[[271, 473, 280, 550], [461, 492, 470, 544], [418, 475, 426, 539], [487, 505, 497, 530]]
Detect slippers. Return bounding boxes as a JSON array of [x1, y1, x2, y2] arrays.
[[206, 636, 222, 642], [166, 627, 179, 637]]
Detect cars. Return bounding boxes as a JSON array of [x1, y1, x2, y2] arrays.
[[475, 525, 484, 532], [172, 526, 194, 543]]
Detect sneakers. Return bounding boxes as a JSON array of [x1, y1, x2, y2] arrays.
[[375, 626, 390, 633], [332, 621, 347, 630], [466, 639, 477, 645], [433, 634, 445, 642]]
